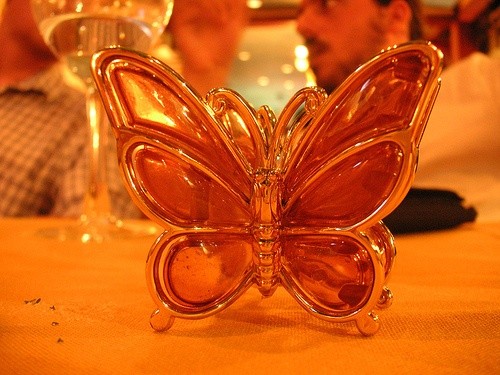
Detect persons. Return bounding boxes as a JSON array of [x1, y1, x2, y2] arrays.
[[0, 0, 141, 218], [170, 0, 500, 231]]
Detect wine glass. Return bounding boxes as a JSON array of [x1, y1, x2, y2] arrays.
[[31, 0, 176, 243]]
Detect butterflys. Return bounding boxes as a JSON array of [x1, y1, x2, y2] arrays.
[[91, 40, 443, 336]]
